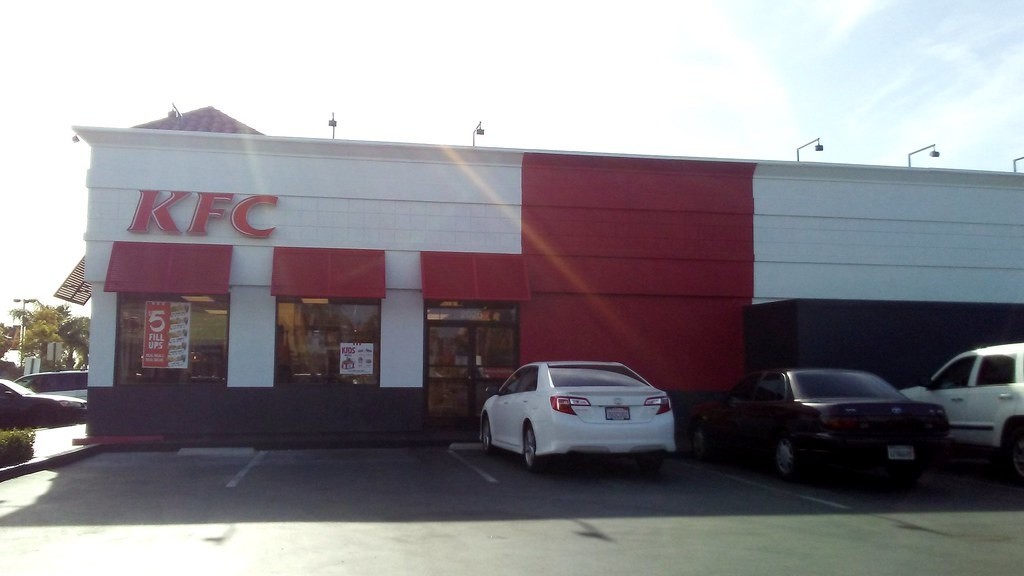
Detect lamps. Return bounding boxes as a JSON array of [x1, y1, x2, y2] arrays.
[[472, 120, 485, 146], [167, 103, 183, 130], [907, 143, 939, 167], [328, 112, 337, 139], [796, 138, 823, 162]]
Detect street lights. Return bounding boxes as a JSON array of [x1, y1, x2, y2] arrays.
[[12, 297, 31, 376]]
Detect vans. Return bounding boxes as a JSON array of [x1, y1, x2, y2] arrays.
[[5, 368, 88, 403]]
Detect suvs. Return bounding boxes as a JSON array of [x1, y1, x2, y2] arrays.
[[894, 342, 1024, 491]]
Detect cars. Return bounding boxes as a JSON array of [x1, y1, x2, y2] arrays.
[[0, 377, 87, 429], [476, 361, 679, 474], [687, 367, 950, 487]]
